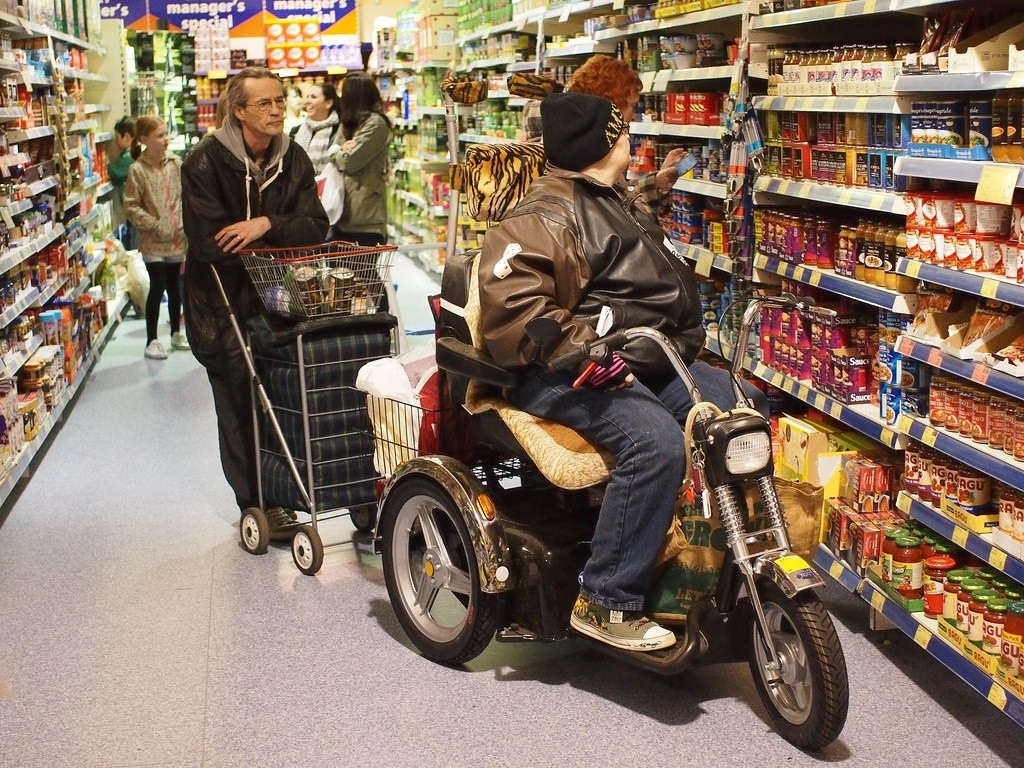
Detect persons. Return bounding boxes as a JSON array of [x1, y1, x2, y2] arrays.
[[563, 54, 690, 216], [180, 66, 330, 540], [107, 114, 138, 251], [322, 70, 394, 314], [122, 115, 191, 359], [283, 84, 308, 135], [478, 93, 770, 652], [289, 82, 342, 177]]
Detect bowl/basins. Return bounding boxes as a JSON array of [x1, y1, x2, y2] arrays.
[[660, 32, 724, 69]]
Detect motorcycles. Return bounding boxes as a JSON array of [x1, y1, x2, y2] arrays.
[[351, 239, 850, 753]]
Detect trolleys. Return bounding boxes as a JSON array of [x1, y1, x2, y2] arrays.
[[211, 239, 405, 575]]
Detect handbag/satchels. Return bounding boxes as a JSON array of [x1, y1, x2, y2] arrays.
[[314, 161, 345, 227], [641, 402, 826, 626], [126, 250, 151, 317]]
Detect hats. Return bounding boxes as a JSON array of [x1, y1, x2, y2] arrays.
[[540, 92, 624, 173]]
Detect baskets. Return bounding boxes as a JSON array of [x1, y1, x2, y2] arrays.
[[239, 240, 398, 323], [347, 384, 531, 489]]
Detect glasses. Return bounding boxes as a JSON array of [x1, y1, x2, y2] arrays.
[[242, 95, 288, 112]]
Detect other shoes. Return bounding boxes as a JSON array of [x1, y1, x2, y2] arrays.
[[266, 506, 304, 540]]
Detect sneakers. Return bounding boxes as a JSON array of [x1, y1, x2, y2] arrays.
[[171, 333, 190, 349], [569, 591, 677, 651], [144, 338, 168, 359]]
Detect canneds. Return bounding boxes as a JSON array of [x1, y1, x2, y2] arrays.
[[759, 108, 951, 191], [21, 343, 66, 403], [767, 41, 915, 95], [879, 521, 1024, 680], [660, 189, 747, 347], [903, 374, 1024, 543], [293, 267, 356, 317], [909, 99, 993, 154], [760, 206, 919, 293], [635, 93, 668, 122], [652, 141, 727, 182]]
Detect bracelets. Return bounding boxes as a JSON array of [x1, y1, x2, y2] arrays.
[[656, 185, 669, 194]]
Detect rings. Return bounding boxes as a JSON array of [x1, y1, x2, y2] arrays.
[[237, 236, 241, 240]]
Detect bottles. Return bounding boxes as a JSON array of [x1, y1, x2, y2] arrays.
[[761, 42, 918, 294]]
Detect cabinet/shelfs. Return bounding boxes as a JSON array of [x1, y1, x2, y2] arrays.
[[0, 1, 1024, 724]]
